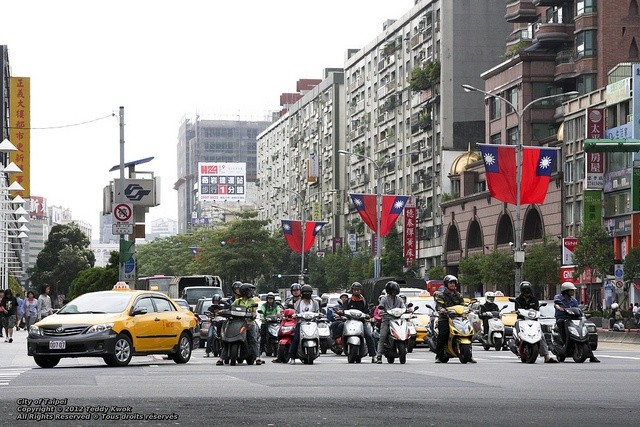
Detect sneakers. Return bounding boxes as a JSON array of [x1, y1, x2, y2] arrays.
[[9, 337, 13, 342], [216, 359, 223, 365], [290, 359, 296, 364], [271, 358, 279, 362], [203, 353, 209, 357], [502, 346, 510, 350], [5, 336, 9, 341], [256, 359, 262, 364], [372, 356, 377, 362], [377, 354, 382, 363]]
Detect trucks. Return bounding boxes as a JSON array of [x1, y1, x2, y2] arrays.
[[278, 288, 319, 303], [169, 274, 224, 313], [361, 275, 431, 304]]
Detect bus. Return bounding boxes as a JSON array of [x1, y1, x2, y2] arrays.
[[136, 275, 175, 295]]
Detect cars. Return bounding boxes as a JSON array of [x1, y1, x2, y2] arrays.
[[403, 290, 436, 347], [472, 290, 517, 340], [537, 299, 598, 354], [26, 281, 198, 369]]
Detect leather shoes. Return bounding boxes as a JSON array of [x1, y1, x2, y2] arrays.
[[590, 356, 600, 361], [468, 358, 477, 362], [435, 357, 441, 362]]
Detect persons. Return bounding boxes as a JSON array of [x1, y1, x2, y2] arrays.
[[515, 280, 559, 363], [628, 303, 633, 314], [16, 293, 23, 330], [374, 294, 386, 329], [285, 298, 291, 309], [335, 282, 379, 363], [290, 283, 321, 365], [259, 292, 283, 357], [400, 296, 414, 322], [272, 282, 303, 363], [578, 301, 585, 310], [318, 296, 334, 323], [19, 292, 40, 333], [433, 291, 442, 302], [203, 293, 224, 357], [638, 305, 640, 314], [63, 300, 70, 306], [333, 294, 350, 345], [600, 302, 603, 312], [434, 274, 478, 363], [224, 281, 265, 365], [376, 281, 407, 363], [607, 303, 624, 331], [215, 282, 263, 366], [0, 289, 4, 337], [553, 281, 600, 362], [36, 283, 53, 321], [1, 289, 18, 343], [633, 303, 639, 314], [478, 291, 509, 351]]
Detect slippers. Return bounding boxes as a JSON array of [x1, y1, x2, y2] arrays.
[[544, 357, 559, 362]]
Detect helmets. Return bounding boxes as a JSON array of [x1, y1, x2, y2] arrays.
[[266, 292, 275, 302], [433, 290, 441, 299], [351, 281, 362, 295], [212, 293, 222, 303], [519, 280, 533, 295], [485, 291, 495, 303], [314, 297, 322, 305], [385, 280, 400, 295], [561, 281, 577, 297], [611, 302, 619, 309], [290, 282, 301, 296], [231, 280, 243, 296], [239, 282, 257, 297], [301, 284, 313, 294], [378, 294, 385, 303], [443, 274, 458, 290], [340, 293, 348, 298], [399, 294, 406, 303]]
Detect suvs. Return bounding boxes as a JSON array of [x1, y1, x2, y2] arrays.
[[194, 296, 230, 348], [173, 298, 190, 310]]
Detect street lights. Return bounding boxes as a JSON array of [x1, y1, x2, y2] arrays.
[[461, 77, 576, 291], [332, 145, 423, 287], [275, 184, 327, 283]]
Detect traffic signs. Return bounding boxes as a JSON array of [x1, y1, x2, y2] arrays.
[[111, 205, 133, 221]]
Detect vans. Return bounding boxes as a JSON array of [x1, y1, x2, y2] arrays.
[[321, 292, 366, 310]]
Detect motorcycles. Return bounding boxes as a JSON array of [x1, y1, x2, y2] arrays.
[[327, 300, 347, 355], [423, 304, 439, 354], [315, 316, 331, 355], [401, 306, 419, 352], [436, 298, 477, 363], [217, 299, 259, 366], [372, 303, 413, 363], [334, 300, 375, 363], [203, 311, 227, 357], [286, 302, 327, 365], [275, 301, 298, 364], [507, 298, 547, 364], [551, 306, 589, 363], [477, 303, 508, 351], [257, 310, 281, 357]]
